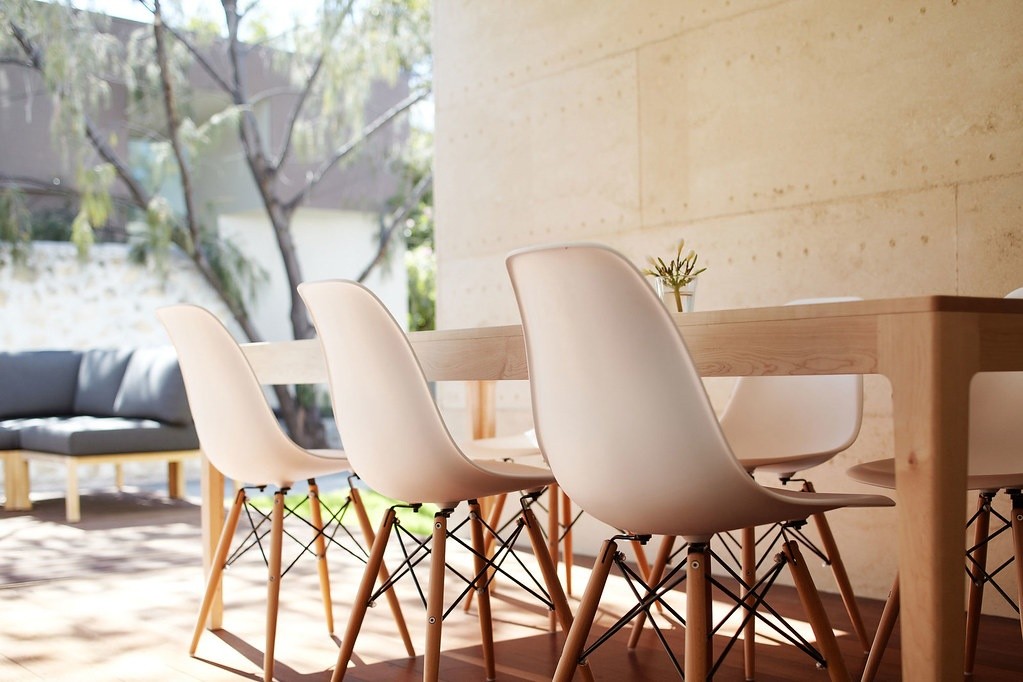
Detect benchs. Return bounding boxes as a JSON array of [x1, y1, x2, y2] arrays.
[[0, 347, 200, 523]]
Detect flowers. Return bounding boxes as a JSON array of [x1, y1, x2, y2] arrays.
[[641, 239, 707, 313]]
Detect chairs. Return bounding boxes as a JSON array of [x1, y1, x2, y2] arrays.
[[156, 241, 871, 682], [847, 285, 1023, 682]]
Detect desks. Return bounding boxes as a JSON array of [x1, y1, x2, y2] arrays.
[[202, 296, 1023, 682]]
